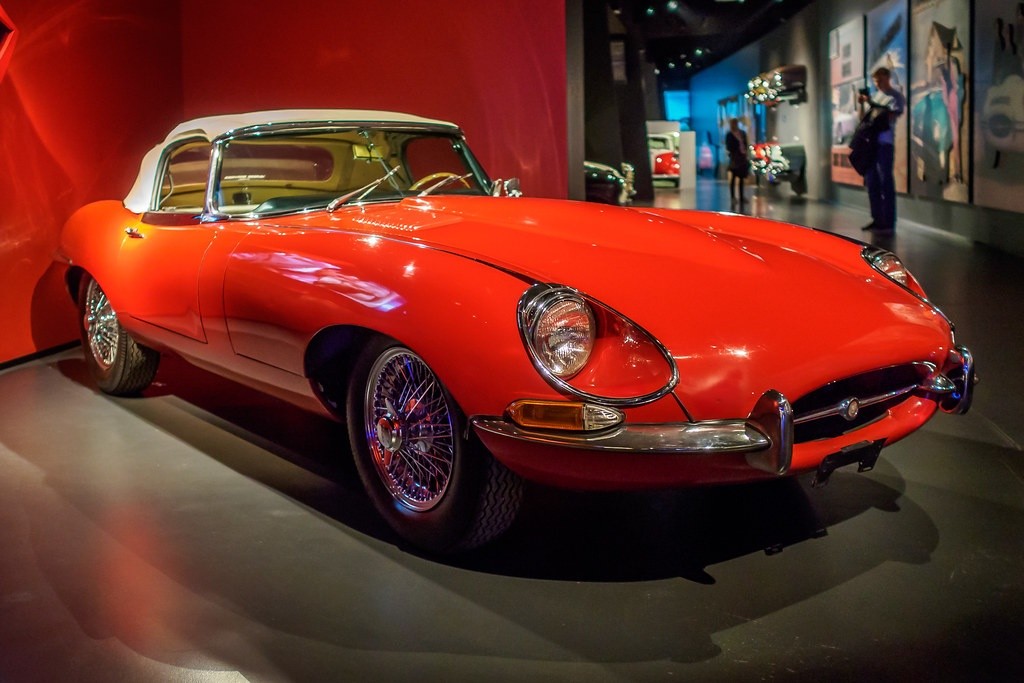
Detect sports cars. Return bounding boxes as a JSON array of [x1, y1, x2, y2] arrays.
[[56, 108, 982, 553]]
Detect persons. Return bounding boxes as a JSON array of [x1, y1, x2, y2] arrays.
[[858, 67, 906, 231], [726, 118, 749, 203]]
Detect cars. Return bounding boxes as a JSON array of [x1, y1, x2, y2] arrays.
[[646, 133, 680, 189]]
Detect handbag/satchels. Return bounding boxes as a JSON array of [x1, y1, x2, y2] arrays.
[[848, 116, 877, 176]]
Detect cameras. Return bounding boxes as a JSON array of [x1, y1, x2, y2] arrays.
[[860, 88, 869, 96]]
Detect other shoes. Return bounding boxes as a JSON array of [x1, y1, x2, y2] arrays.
[[739, 197, 749, 204], [862, 220, 895, 233], [731, 196, 737, 204]]
